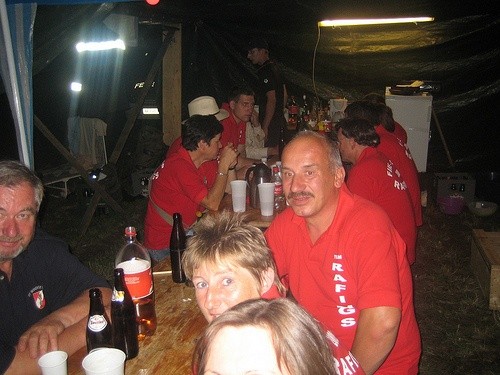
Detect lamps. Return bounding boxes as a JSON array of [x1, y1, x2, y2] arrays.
[[318, 16, 434, 27]]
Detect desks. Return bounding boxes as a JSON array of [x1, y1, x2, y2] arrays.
[[201, 195, 280, 228], [66, 256, 208, 375]]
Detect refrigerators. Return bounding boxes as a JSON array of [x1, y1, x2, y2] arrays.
[[385, 86, 433, 173]]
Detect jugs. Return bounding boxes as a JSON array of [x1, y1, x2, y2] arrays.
[[244, 163, 271, 209]]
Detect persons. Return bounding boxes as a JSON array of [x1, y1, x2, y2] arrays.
[[244, 36, 288, 146], [0, 88, 422, 375]]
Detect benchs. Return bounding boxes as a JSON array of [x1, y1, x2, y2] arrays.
[[36, 163, 89, 198]]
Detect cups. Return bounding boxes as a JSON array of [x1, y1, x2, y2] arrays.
[[229, 180, 247, 213], [257, 182, 274, 216], [82, 347, 126, 375], [38, 350, 68, 375]]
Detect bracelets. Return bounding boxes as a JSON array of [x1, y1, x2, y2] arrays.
[[217, 173, 227, 176], [229, 168, 234, 170]]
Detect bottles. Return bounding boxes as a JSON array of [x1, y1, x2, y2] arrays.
[[287, 96, 332, 134], [86, 288, 114, 353], [114, 227, 157, 340], [270, 160, 285, 211], [111, 268, 139, 360], [170, 213, 186, 283]]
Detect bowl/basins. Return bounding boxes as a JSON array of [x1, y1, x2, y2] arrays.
[[437, 197, 464, 214], [468, 201, 497, 215]]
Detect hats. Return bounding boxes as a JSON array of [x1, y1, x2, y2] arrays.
[[248, 38, 269, 49], [182, 95, 230, 125]]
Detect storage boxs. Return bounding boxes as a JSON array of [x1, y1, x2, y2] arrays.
[[429, 170, 476, 206], [472, 228, 500, 312]]
